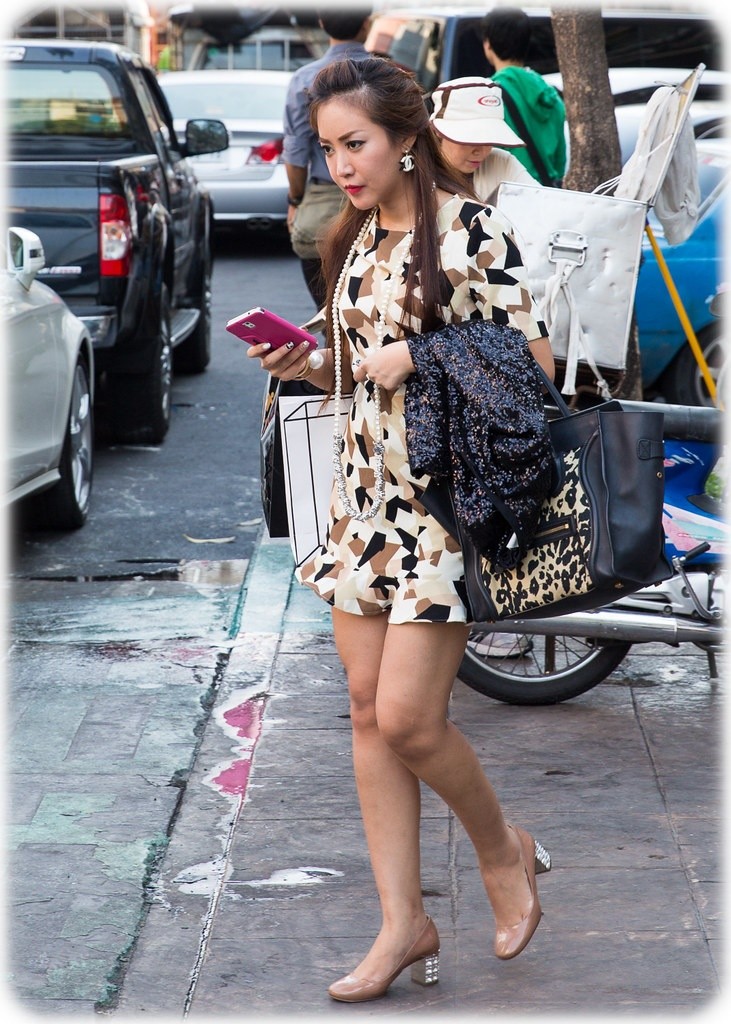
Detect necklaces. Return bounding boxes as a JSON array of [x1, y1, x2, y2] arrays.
[[332, 180, 436, 522]]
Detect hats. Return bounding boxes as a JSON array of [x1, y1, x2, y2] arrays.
[[426, 75, 528, 147]]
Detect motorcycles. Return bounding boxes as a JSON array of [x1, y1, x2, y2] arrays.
[[456, 292, 730, 707]]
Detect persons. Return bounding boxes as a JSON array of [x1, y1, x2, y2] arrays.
[[247, 58, 555, 1003], [281, 0, 375, 337], [480, 6, 567, 188], [428, 77, 544, 203]]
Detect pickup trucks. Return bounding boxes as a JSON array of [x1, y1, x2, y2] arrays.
[[1, 36, 230, 448]]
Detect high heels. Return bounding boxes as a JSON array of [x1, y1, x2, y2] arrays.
[[492, 824, 550, 960], [328, 914, 440, 1003]]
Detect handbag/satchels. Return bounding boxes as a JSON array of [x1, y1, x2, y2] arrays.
[[419, 357, 674, 619], [290, 181, 354, 259]]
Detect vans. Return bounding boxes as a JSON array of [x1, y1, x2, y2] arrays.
[[363, 2, 728, 121]]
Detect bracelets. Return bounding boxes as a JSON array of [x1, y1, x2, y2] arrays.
[[287, 194, 301, 206], [292, 352, 324, 381]]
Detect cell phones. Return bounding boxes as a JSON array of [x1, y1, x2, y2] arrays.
[[225, 307, 318, 354]]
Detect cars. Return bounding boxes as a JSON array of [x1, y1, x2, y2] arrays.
[[1, 225, 97, 536], [154, 68, 312, 259], [541, 66, 730, 410]]
